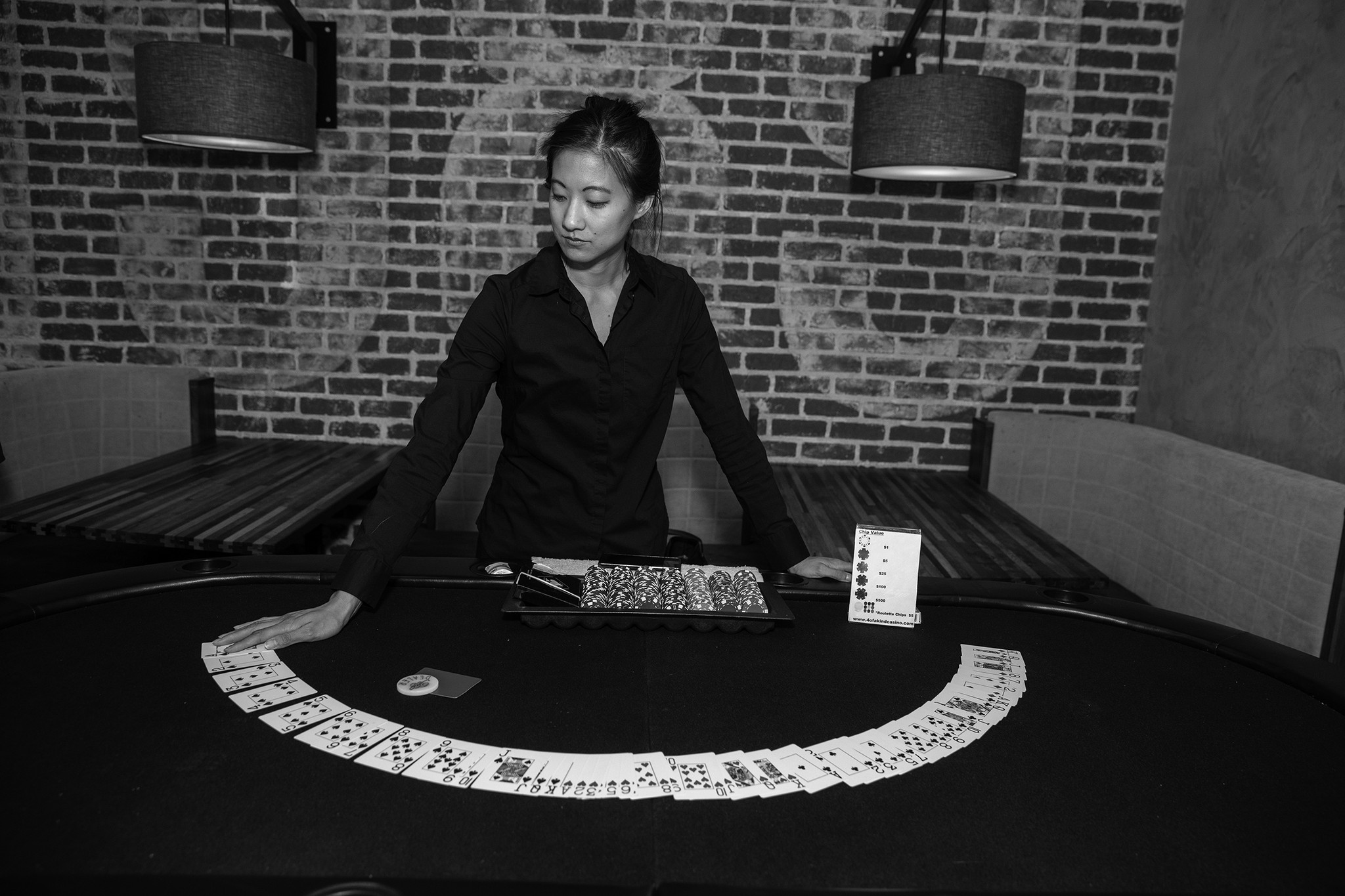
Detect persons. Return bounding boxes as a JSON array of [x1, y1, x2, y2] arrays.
[[209, 92, 850, 658]]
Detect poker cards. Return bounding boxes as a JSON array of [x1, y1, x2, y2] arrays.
[[199, 640, 1027, 802]]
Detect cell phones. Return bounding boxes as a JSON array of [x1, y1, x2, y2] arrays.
[[596, 552, 682, 570]]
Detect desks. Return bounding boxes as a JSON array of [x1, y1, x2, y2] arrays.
[[770, 460, 1107, 597], [3, 556, 1345, 894], [0, 436, 408, 560]]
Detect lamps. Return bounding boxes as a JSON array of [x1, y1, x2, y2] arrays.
[[851, 0, 1025, 184], [133, 3, 319, 158]]
